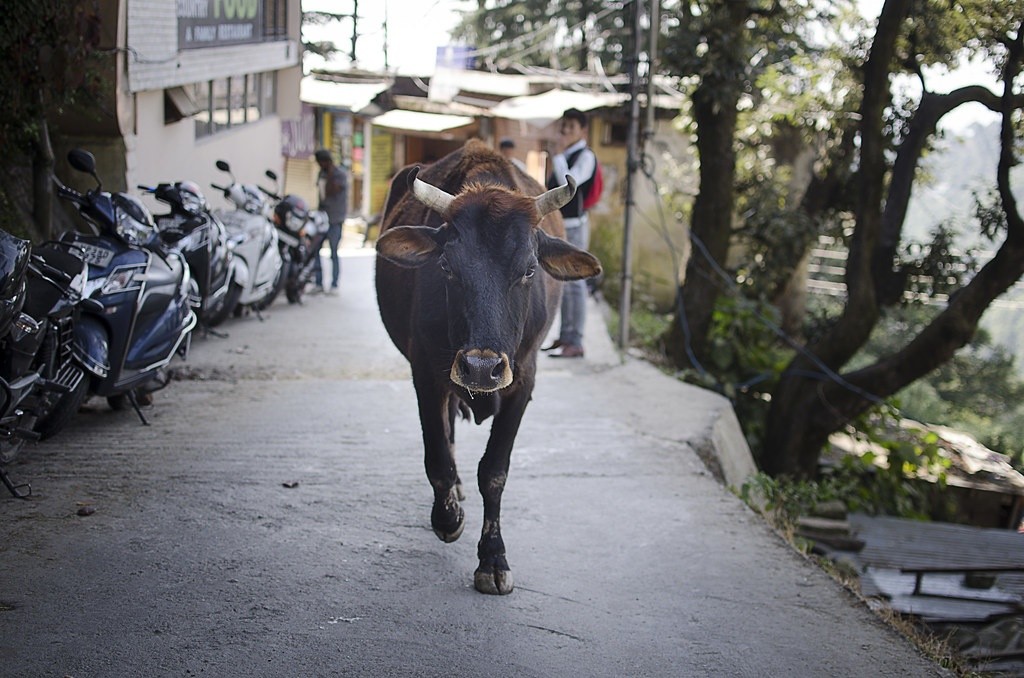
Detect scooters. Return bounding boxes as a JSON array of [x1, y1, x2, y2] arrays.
[[0, 148, 332, 499]]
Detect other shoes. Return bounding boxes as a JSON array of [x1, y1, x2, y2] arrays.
[[540, 339, 563, 351], [548, 344, 583, 358], [308, 286, 323, 294], [329, 286, 337, 296]]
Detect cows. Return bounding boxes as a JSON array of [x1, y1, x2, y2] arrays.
[[375, 137, 604, 596]]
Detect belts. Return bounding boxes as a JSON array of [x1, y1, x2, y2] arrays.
[[563, 212, 589, 229]]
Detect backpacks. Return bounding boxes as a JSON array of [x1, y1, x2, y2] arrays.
[[572, 147, 602, 209]]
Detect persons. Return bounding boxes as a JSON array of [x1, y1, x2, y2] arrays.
[[547, 107, 605, 359], [307, 150, 349, 295], [496, 139, 526, 176]]
